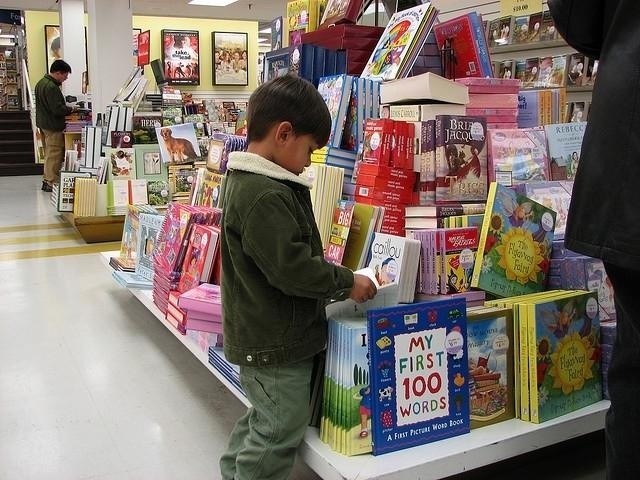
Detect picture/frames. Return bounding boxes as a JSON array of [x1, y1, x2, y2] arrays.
[[160, 29, 200, 86], [137, 29, 151, 66], [212, 31, 248, 86], [133, 29, 145, 76], [58, 170, 93, 214], [136, 145, 167, 181], [43, 24, 89, 88]]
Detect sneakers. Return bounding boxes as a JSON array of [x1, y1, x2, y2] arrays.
[[42, 182, 48, 190], [45, 185, 51, 191]]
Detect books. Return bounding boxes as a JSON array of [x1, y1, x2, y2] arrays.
[[0, 46, 23, 113], [49, 6, 618, 463]]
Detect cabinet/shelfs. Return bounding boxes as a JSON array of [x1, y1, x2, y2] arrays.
[[0, 54, 20, 111], [488, 38, 593, 92]]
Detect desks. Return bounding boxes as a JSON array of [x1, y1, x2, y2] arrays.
[[63, 130, 83, 155]]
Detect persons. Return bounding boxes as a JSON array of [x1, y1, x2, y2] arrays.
[[215, 74, 379, 479], [544, 0, 639, 480], [33, 59, 81, 193]]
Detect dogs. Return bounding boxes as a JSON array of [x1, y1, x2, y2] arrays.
[[160, 128, 198, 163]]
